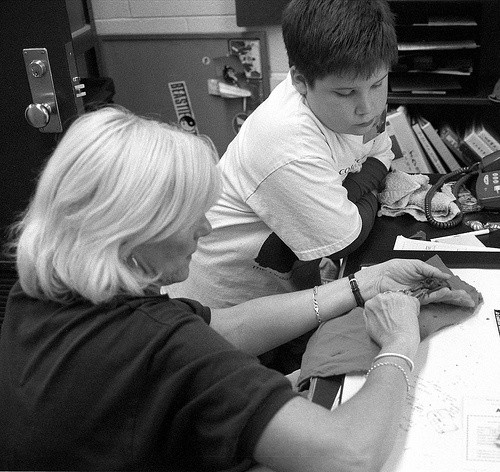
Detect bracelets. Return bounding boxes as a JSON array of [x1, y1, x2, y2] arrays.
[[348, 273, 366, 308], [373, 354, 414, 373], [366, 363, 412, 392], [310, 284, 322, 326]]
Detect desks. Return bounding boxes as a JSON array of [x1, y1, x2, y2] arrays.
[[308, 210, 500, 411]]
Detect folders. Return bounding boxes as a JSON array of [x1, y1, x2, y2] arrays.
[[411, 115, 500, 174]]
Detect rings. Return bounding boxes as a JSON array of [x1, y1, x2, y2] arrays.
[[383, 288, 412, 295]]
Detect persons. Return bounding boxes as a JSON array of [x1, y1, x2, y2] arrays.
[[0, 108, 475, 472], [159, 0, 399, 373]]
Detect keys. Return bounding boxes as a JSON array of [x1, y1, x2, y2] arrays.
[[411, 277, 445, 298]]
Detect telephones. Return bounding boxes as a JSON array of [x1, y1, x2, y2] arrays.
[[476, 149, 500, 209]]
